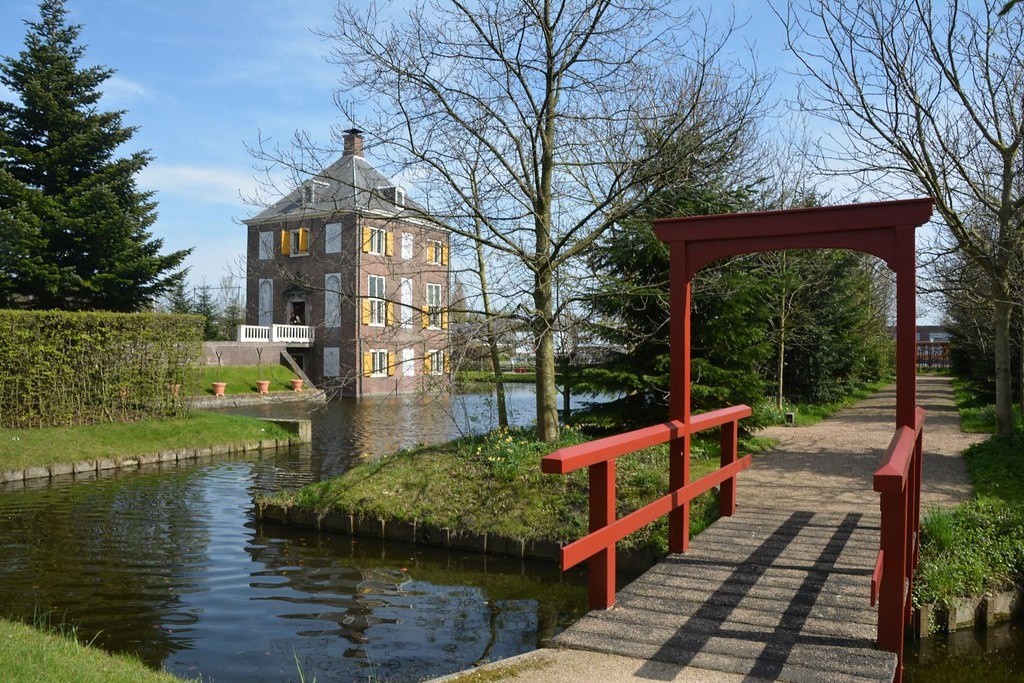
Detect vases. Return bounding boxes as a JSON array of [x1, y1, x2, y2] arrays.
[[171, 384, 181, 398], [256, 381, 270, 393], [290, 379, 303, 392], [212, 382, 227, 396]]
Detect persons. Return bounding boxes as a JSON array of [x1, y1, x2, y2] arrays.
[[290, 313, 301, 325]]
